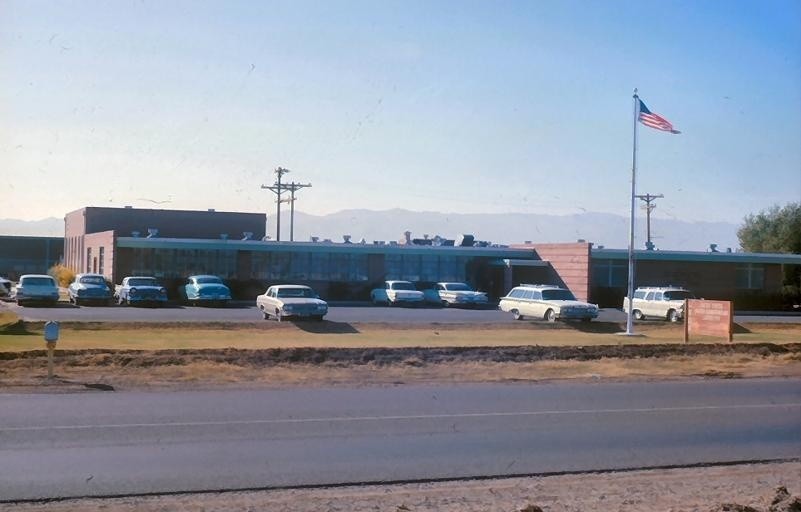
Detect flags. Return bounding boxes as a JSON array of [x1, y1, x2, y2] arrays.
[[637, 99, 682, 134]]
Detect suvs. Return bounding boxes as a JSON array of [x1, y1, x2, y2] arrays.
[[622, 284, 699, 324], [496, 283, 601, 323]]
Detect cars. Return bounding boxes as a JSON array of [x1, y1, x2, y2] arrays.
[[0, 274, 10, 297], [368, 278, 425, 307], [66, 272, 115, 306], [420, 282, 490, 308], [13, 272, 60, 307], [255, 282, 328, 322], [175, 274, 234, 307]]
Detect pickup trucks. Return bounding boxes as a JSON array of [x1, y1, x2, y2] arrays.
[[111, 275, 170, 308]]
